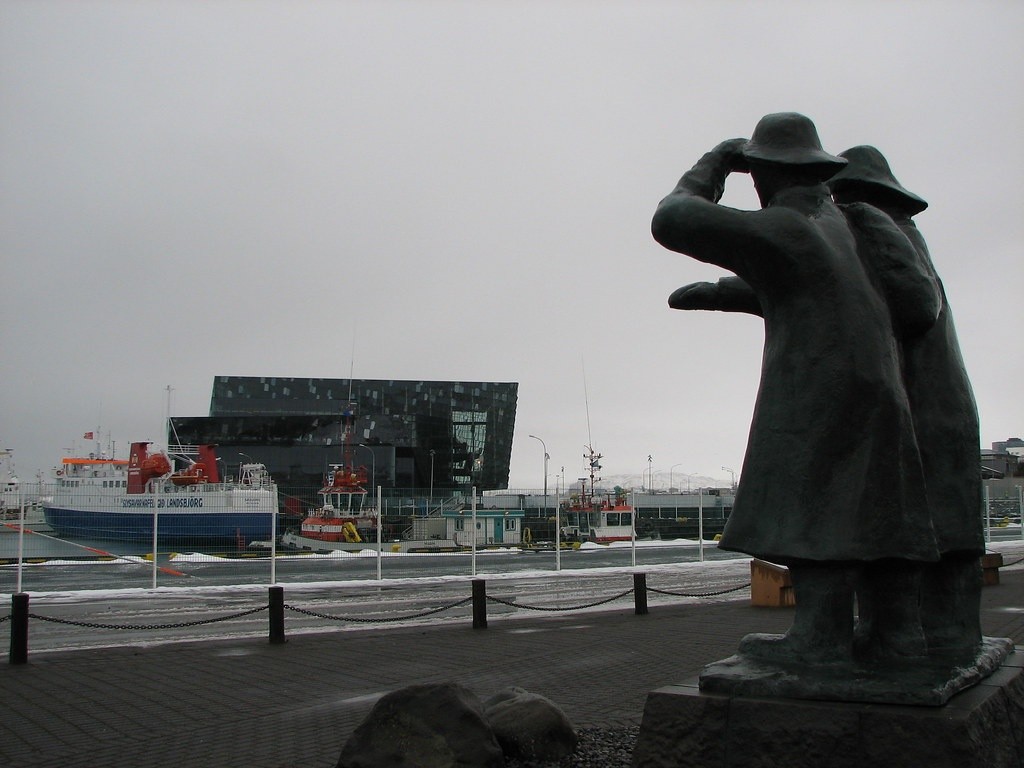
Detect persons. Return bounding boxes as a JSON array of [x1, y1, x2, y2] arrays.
[[651, 111, 986, 669]]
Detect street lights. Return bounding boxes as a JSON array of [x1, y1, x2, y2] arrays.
[[671, 464, 682, 488], [722, 467, 738, 485], [680, 473, 698, 490], [652, 470, 663, 489], [529, 435, 546, 493], [643, 467, 654, 487]]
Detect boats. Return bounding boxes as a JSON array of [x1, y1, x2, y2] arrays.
[[0, 448, 55, 532], [281, 415, 464, 554], [41, 386, 280, 547], [560, 372, 638, 544]]
